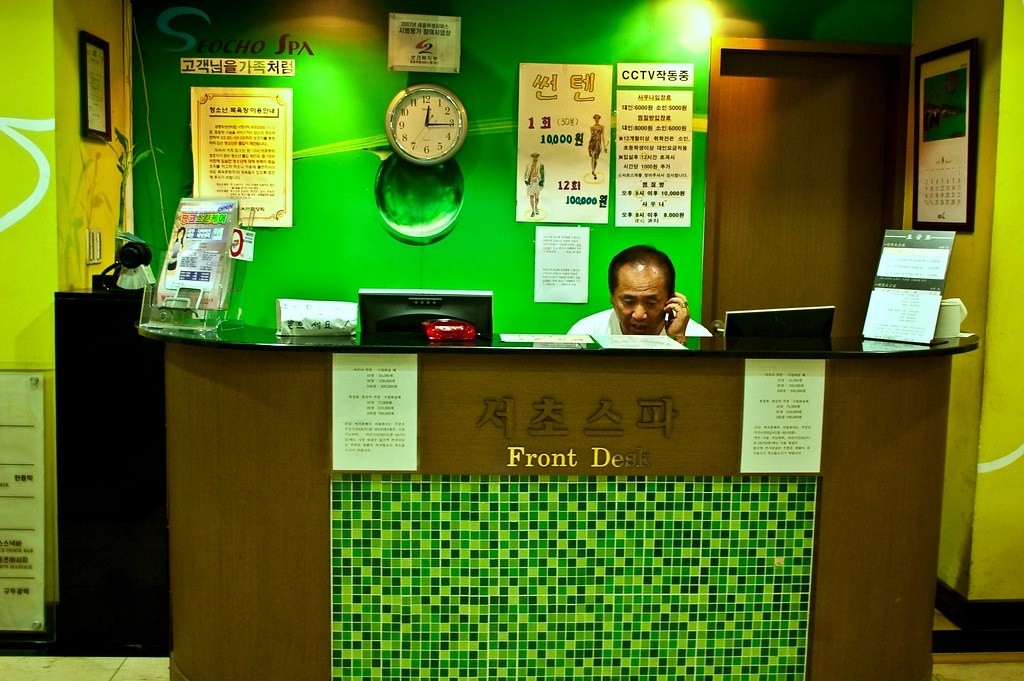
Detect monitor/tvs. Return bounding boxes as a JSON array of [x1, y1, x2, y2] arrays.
[[724, 306, 836, 338], [358, 288, 494, 337]]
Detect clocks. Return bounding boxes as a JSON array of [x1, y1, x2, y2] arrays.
[[385, 83, 468, 167]]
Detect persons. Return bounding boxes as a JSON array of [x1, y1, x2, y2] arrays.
[[524, 153, 545, 217], [588, 114, 607, 180], [167, 227, 189, 276], [567, 245, 714, 337]]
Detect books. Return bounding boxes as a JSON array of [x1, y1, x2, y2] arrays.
[[155, 198, 240, 310]]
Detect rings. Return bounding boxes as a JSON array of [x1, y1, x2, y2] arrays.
[[681, 302, 688, 308]]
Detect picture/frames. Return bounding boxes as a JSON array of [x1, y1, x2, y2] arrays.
[[914, 37, 983, 237]]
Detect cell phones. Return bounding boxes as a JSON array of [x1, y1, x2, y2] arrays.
[[664, 311, 672, 329]]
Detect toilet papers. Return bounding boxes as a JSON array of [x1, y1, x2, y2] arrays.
[[934, 297, 969, 339]]
